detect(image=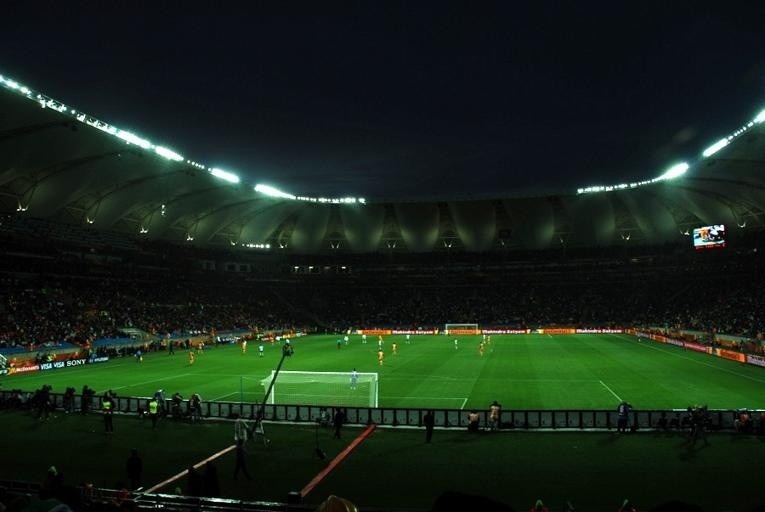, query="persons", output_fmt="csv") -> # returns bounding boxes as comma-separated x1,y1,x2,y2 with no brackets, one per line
377,349,384,365
337,338,341,349
626,252,765,359
378,339,382,350
482,334,486,343
392,342,397,354
487,334,491,343
378,334,384,344
319,261,626,336
0,213,318,374
1,386,764,512
349,368,359,390
479,341,484,357
698,224,725,242
454,338,458,350
362,333,368,344
344,335,349,345
405,333,410,344
